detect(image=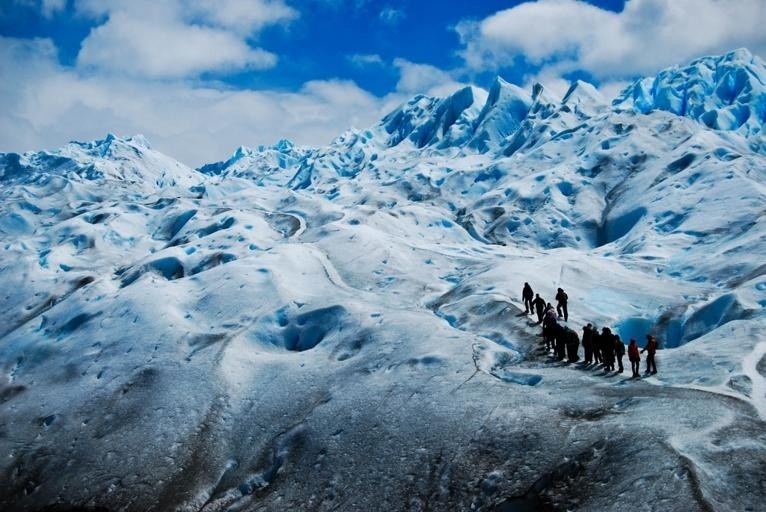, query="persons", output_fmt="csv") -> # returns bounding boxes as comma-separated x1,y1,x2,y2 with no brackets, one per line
627,337,642,378
530,292,546,323
640,334,659,375
535,301,616,372
555,286,569,322
521,280,535,314
614,333,626,373
